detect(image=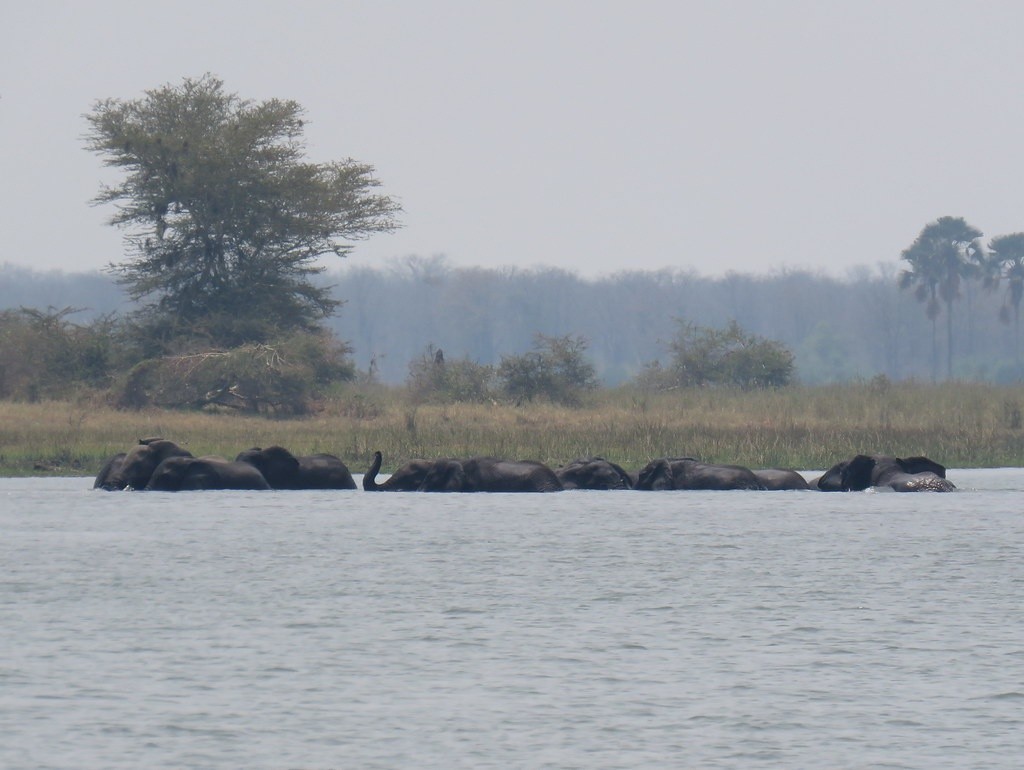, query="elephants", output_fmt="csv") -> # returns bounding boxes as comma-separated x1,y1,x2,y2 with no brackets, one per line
92,437,958,492
